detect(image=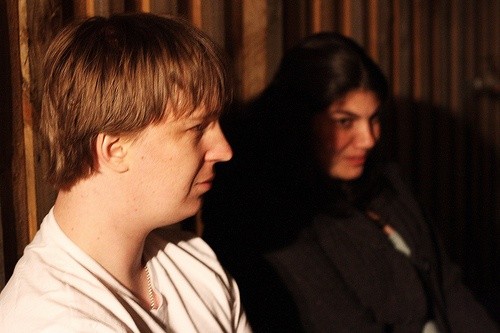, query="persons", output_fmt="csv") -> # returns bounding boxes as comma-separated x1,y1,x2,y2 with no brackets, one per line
200,33,496,333
0,12,253,332
